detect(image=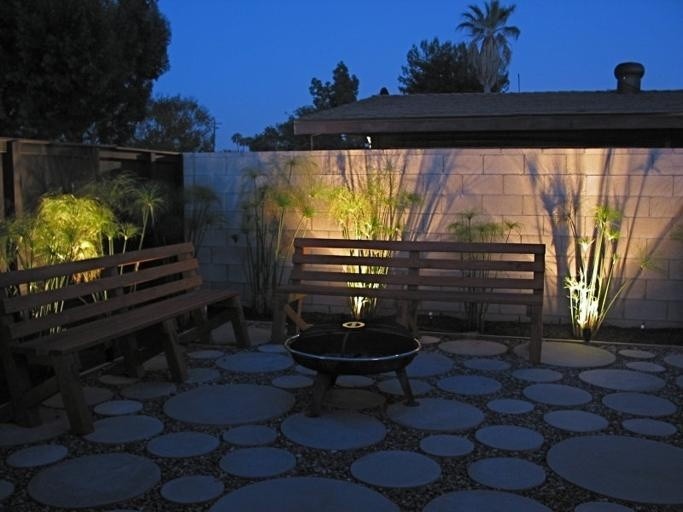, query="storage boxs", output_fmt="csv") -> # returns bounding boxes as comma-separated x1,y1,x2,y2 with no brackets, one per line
0,241,254,438
270,235,551,372
